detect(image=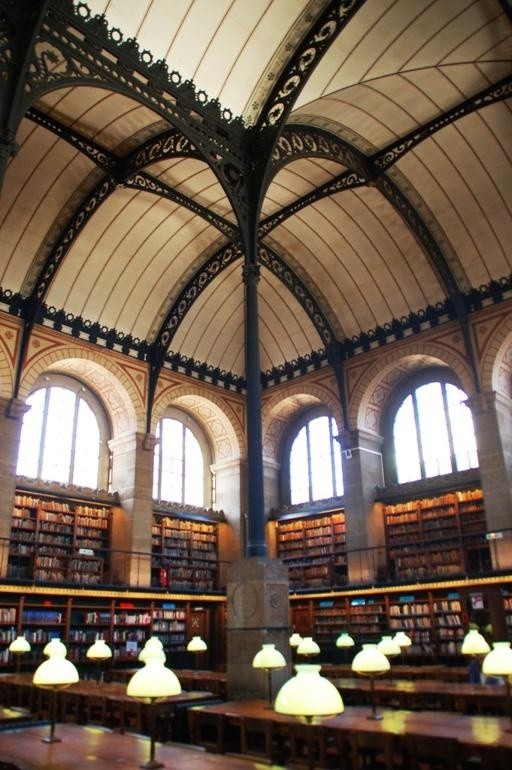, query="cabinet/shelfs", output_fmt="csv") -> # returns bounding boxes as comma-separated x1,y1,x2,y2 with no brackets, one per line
9,491,112,584
275,510,347,588
308,586,512,654
0,594,189,673
150,513,220,589
382,489,491,579
